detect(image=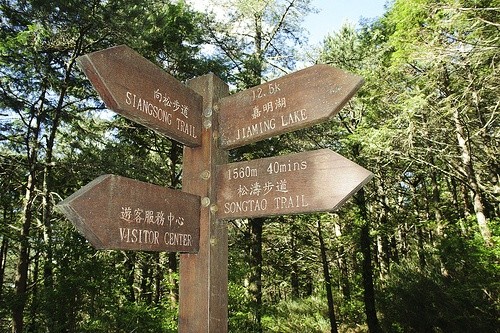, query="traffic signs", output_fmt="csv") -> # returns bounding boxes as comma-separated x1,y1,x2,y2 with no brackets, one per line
56,44,374,253
